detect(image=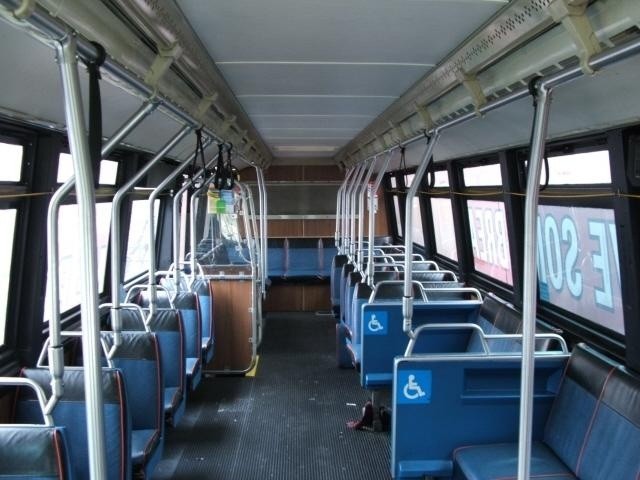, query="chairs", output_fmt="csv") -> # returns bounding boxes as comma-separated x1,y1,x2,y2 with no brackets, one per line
159,261,216,362
82,301,166,466
1,375,73,480
329,236,639,480
149,270,204,382
126,283,187,419
22,330,134,479
